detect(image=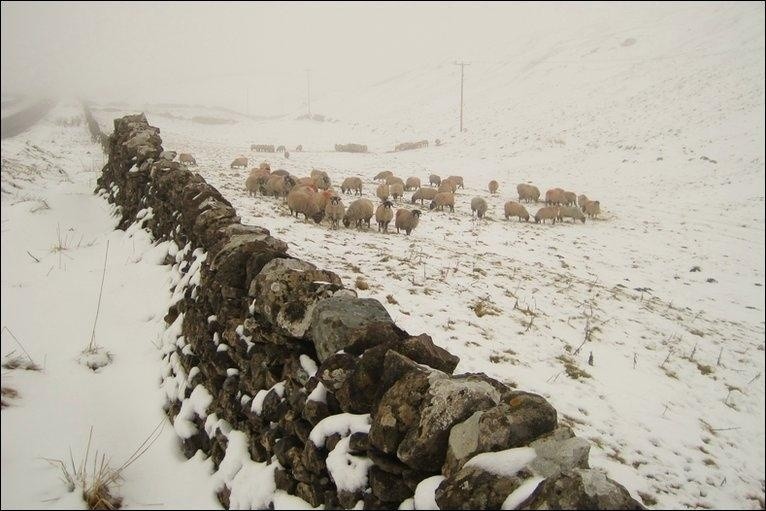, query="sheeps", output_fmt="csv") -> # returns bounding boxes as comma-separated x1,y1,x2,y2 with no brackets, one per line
230,158,248,170
335,143,367,153
471,180,601,225
245,163,373,229
374,171,464,236
251,144,302,159
395,139,441,151
179,154,196,166
